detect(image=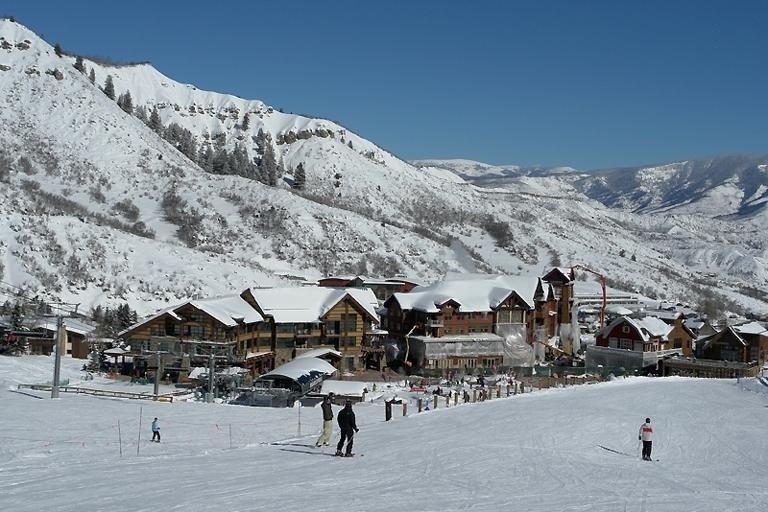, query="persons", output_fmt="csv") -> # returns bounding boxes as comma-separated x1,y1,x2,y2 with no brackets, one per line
151,417,161,443
638,418,655,462
314,394,335,447
335,399,359,457
